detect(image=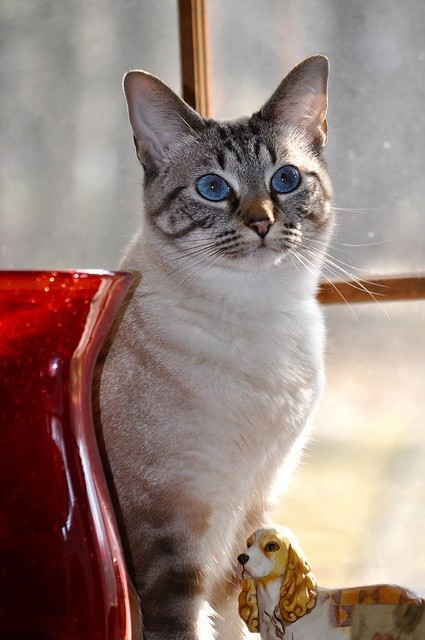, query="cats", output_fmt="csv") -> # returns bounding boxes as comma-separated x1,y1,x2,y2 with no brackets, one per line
95,52,392,640
